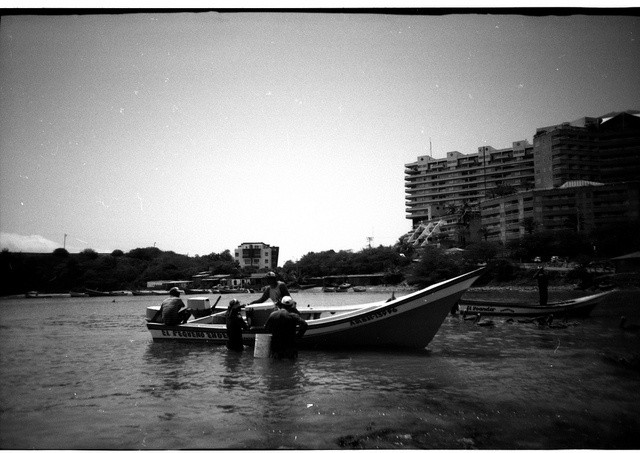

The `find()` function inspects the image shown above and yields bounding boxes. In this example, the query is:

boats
[447,287,620,321]
[145,263,486,354]
[323,282,366,291]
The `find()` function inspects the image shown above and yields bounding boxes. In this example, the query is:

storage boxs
[187,298,210,311]
[245,305,278,326]
[146,306,162,321]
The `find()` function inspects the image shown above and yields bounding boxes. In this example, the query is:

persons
[161,287,191,327]
[264,296,308,362]
[247,271,301,315]
[224,300,250,351]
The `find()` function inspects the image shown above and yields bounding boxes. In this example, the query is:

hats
[265,271,276,276]
[229,297,241,307]
[170,286,184,292]
[280,295,293,305]
